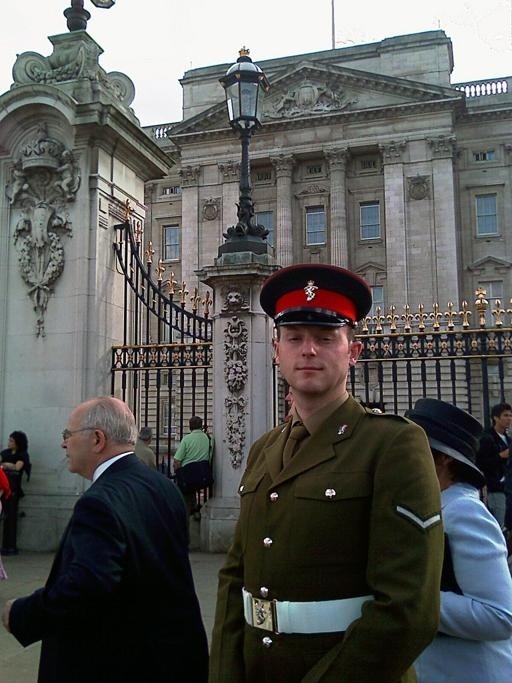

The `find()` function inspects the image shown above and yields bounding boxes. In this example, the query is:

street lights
[219,45,277,257]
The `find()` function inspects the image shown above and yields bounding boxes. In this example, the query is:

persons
[135,427,156,469]
[2,396,210,683]
[285,385,295,407]
[0,467,12,516]
[477,403,512,529]
[174,416,214,515]
[209,263,444,683]
[403,398,512,683]
[0,431,29,555]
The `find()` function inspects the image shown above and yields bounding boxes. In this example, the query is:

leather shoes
[0,548,16,555]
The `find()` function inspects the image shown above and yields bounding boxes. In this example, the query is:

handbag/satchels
[440,533,464,597]
[5,470,24,500]
[175,461,215,494]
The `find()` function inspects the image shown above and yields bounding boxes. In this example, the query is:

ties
[282,425,309,469]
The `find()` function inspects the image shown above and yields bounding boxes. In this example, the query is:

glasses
[63,427,108,442]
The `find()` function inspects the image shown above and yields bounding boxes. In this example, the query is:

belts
[242,587,375,633]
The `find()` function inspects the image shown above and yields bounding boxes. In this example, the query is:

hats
[260,264,372,329]
[404,398,484,476]
